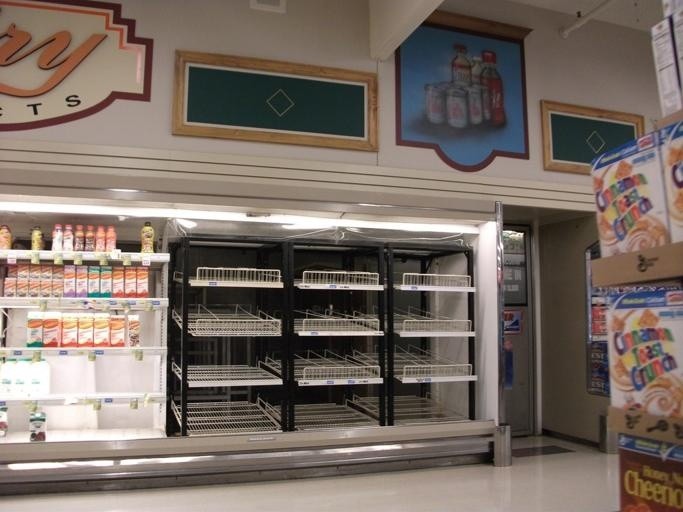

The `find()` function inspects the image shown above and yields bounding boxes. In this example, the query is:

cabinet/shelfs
[289,239,385,431]
[0,250,170,463]
[168,234,288,436]
[385,242,475,426]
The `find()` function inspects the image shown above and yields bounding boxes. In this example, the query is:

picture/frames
[172,49,378,151]
[541,100,644,175]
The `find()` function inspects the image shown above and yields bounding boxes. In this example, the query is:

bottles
[0,219,155,254]
[452,42,506,129]
